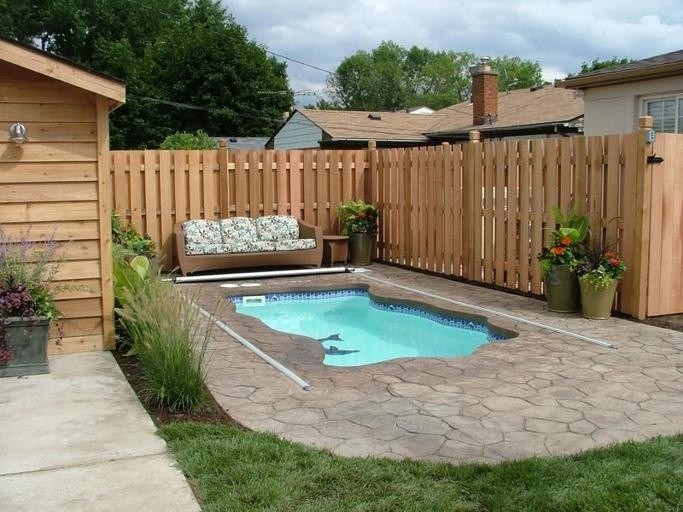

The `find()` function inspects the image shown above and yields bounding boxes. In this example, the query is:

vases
[0,314,52,378]
[347,230,374,264]
[544,258,617,320]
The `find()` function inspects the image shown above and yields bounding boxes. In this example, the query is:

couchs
[173,216,325,275]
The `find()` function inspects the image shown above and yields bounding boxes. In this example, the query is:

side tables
[323,235,350,267]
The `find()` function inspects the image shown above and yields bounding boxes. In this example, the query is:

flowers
[338,198,380,234]
[0,226,69,360]
[538,197,626,289]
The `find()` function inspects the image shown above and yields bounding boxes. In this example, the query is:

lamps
[7,123,27,144]
